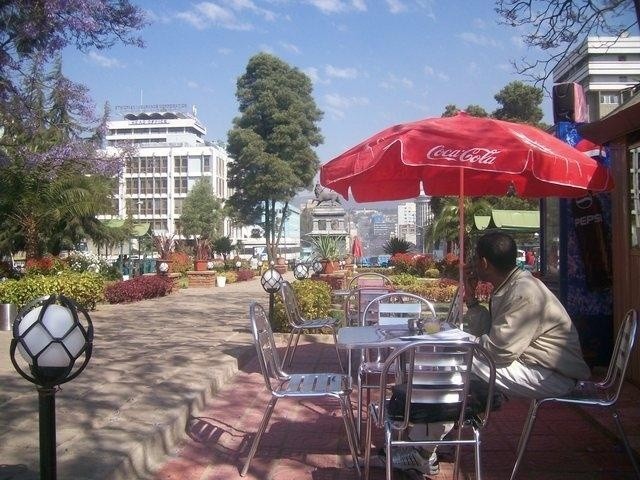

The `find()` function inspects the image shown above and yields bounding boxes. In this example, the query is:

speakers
[552,81,585,124]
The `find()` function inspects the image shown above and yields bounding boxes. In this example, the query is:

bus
[213,236,302,269]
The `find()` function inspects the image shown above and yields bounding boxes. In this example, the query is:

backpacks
[388,369,505,422]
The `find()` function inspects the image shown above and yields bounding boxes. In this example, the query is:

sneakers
[377,445,441,476]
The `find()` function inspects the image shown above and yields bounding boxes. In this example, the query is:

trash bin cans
[0,303,16,331]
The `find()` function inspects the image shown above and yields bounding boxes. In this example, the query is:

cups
[407,318,421,331]
[426,317,440,333]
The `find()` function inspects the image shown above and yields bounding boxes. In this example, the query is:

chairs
[347,272,392,290]
[279,280,345,374]
[510,309,637,480]
[364,339,496,480]
[344,287,402,326]
[363,292,437,406]
[239,302,363,478]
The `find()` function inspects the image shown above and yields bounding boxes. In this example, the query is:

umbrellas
[319,111,617,331]
[353,238,362,272]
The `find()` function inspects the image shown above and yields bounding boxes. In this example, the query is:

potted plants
[146,232,179,274]
[301,229,349,274]
[193,238,211,272]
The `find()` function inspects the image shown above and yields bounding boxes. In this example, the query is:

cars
[127,255,138,259]
[357,254,391,269]
[516,249,526,263]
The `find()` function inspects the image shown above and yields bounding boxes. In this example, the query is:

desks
[335,287,388,296]
[339,323,468,467]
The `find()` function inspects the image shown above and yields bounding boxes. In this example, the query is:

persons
[380,233,591,475]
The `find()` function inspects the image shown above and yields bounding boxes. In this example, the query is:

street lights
[110,201,145,219]
[408,222,425,254]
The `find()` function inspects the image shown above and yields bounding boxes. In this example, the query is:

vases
[215,275,227,288]
